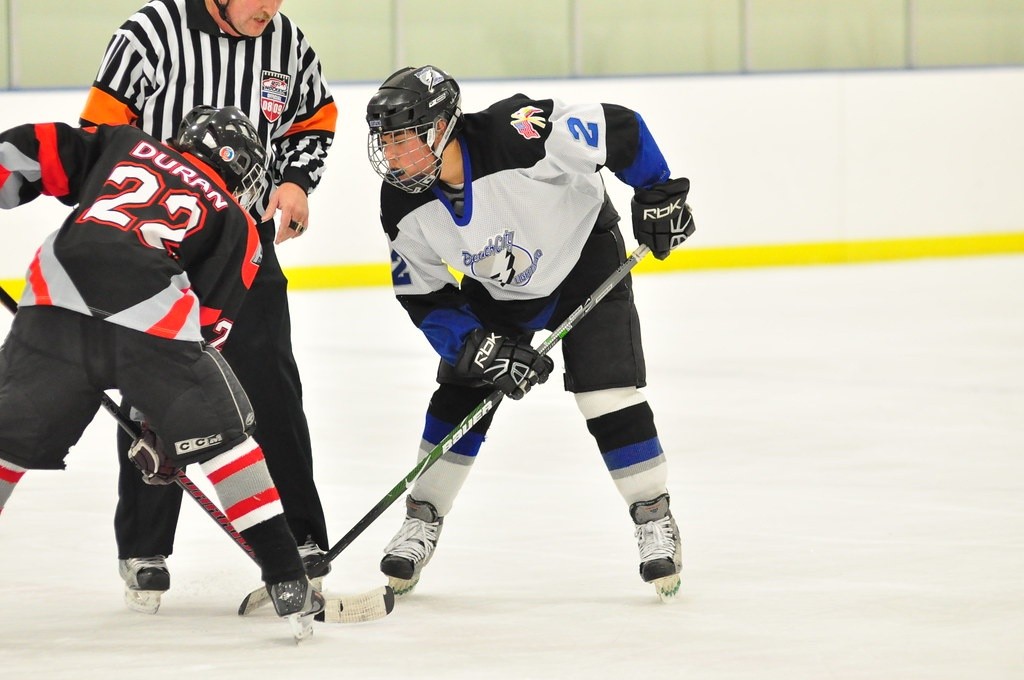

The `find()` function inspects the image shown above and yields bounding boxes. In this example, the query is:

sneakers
[294,537,333,591]
[629,492,683,602]
[263,573,325,642]
[118,551,171,613]
[380,494,444,595]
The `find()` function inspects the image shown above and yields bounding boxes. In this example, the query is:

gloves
[126,426,183,484]
[454,328,555,400]
[630,178,696,261]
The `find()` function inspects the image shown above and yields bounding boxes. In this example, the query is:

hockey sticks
[231,239,660,620]
[0,280,402,630]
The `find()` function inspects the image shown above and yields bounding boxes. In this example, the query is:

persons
[2,0,339,643]
[363,64,696,596]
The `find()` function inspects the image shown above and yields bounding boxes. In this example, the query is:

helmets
[366,65,461,194]
[174,104,267,193]
